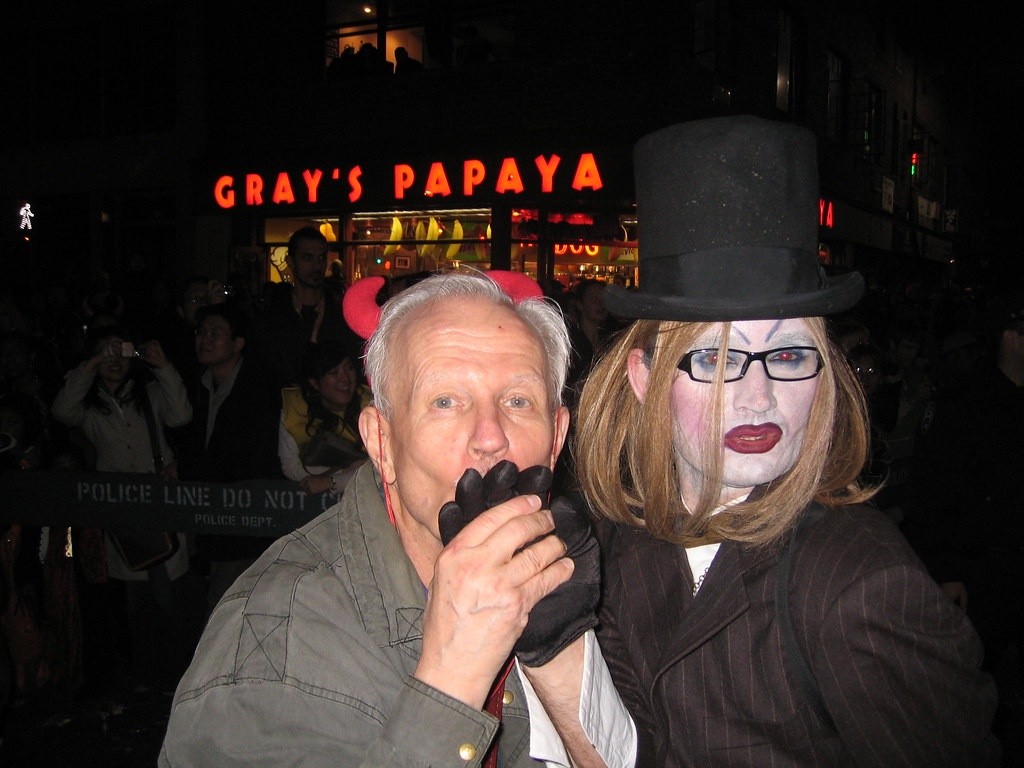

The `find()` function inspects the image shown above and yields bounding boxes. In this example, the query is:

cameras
[121,342,144,356]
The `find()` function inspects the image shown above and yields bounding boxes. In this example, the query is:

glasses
[194,327,233,341]
[188,296,207,304]
[677,346,824,384]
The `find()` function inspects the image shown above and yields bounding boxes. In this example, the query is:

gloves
[438,459,602,668]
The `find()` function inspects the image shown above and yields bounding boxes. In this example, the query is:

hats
[603,115,868,322]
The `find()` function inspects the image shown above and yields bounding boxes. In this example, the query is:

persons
[152,269,572,768]
[438,314,1018,768]
[0,224,1024,768]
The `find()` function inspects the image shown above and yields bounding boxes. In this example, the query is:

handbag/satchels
[0,544,42,666]
[108,527,180,571]
